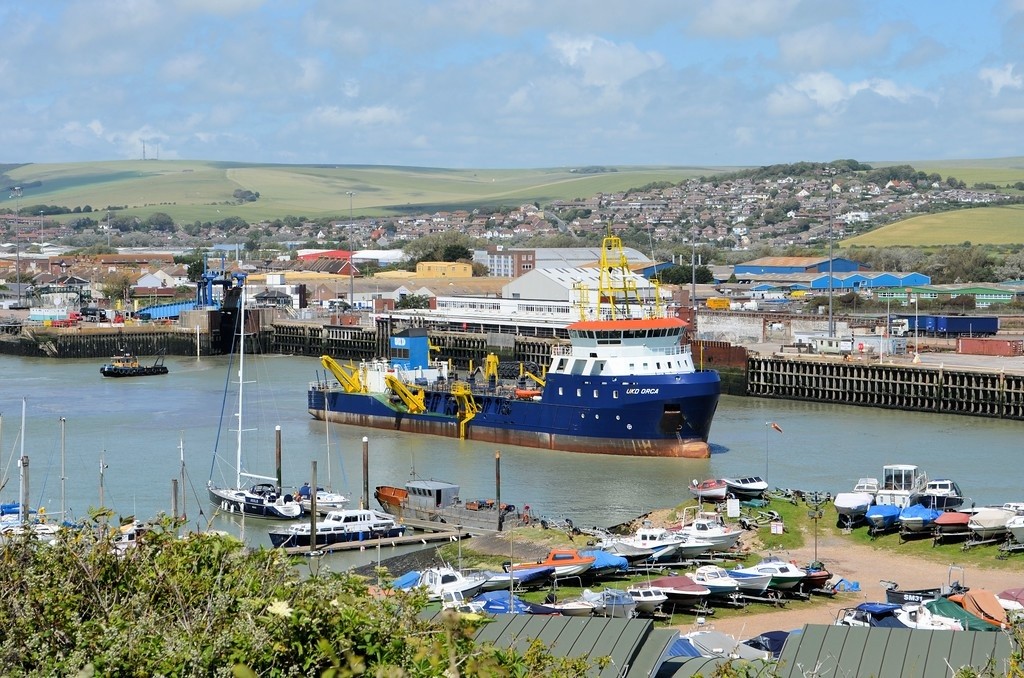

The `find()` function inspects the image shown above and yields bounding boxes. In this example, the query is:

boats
[688,476,727,503]
[347,463,1024,661]
[265,496,410,549]
[725,472,769,497]
[373,452,544,538]
[100,337,169,377]
[515,388,542,399]
[305,192,722,461]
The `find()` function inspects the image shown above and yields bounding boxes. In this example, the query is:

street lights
[9,185,25,307]
[807,511,826,561]
[106,209,112,249]
[345,190,356,309]
[39,210,46,253]
[821,166,842,337]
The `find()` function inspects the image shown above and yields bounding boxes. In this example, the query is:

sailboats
[290,396,351,516]
[0,396,189,562]
[206,284,306,525]
